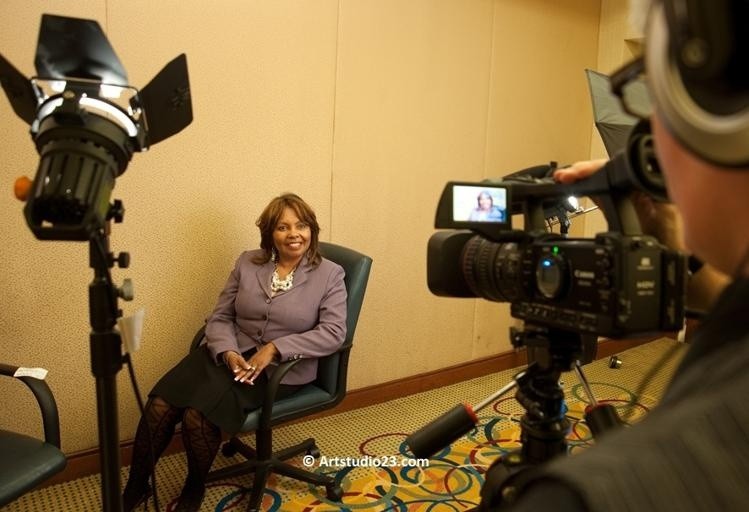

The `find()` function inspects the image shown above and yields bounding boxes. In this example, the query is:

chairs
[191,242,372,512]
[0,362,68,511]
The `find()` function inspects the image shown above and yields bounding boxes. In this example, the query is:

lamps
[0,13,194,512]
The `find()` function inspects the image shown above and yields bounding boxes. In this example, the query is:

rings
[250,367,256,371]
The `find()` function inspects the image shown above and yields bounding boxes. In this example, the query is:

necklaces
[271,260,297,291]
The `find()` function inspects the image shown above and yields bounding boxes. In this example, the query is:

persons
[468,192,502,221]
[502,1,747,509]
[122,192,347,512]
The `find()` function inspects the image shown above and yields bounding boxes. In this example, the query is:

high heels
[124,483,152,511]
